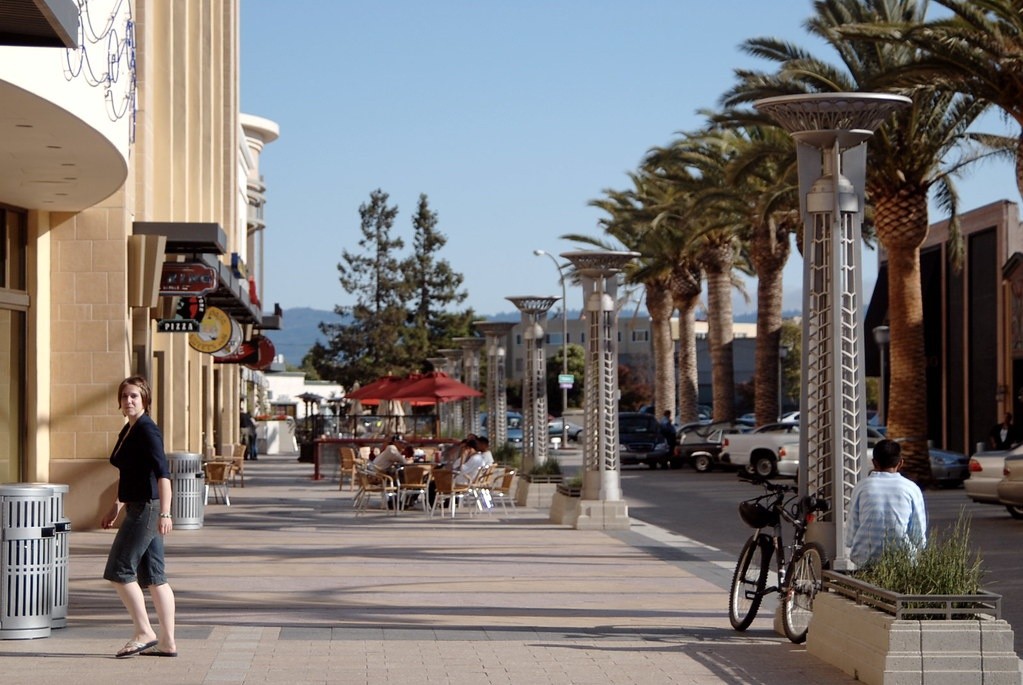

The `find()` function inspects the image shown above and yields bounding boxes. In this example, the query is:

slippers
[115,640,158,657]
[139,645,177,656]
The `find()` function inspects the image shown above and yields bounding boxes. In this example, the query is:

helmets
[740,501,770,529]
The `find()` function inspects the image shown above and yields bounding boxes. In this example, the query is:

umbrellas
[343,369,484,446]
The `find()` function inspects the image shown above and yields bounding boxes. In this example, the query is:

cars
[477,411,585,449]
[617,411,672,471]
[962,441,1023,524]
[641,402,970,491]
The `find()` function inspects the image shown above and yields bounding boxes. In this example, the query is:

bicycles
[729,473,831,644]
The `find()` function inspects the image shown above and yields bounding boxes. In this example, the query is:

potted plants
[804,503,1021,685]
[517,457,565,509]
[549,467,584,524]
[491,441,522,493]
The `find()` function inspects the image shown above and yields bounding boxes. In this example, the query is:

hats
[392,434,406,443]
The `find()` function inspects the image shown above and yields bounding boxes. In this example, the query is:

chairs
[215,444,247,488]
[340,447,519,519]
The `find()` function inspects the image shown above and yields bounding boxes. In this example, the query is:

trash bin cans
[0,480,73,639]
[163,453,207,531]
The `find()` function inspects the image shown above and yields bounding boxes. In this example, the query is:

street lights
[533,248,568,448]
[778,344,789,420]
[872,325,891,428]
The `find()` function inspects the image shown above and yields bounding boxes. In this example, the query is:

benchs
[203,461,232,506]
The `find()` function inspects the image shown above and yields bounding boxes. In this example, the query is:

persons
[240,407,260,460]
[368,432,494,507]
[993,412,1016,451]
[101,377,178,657]
[661,410,675,433]
[844,439,927,570]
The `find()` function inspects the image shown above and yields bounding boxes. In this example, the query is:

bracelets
[159,513,172,518]
